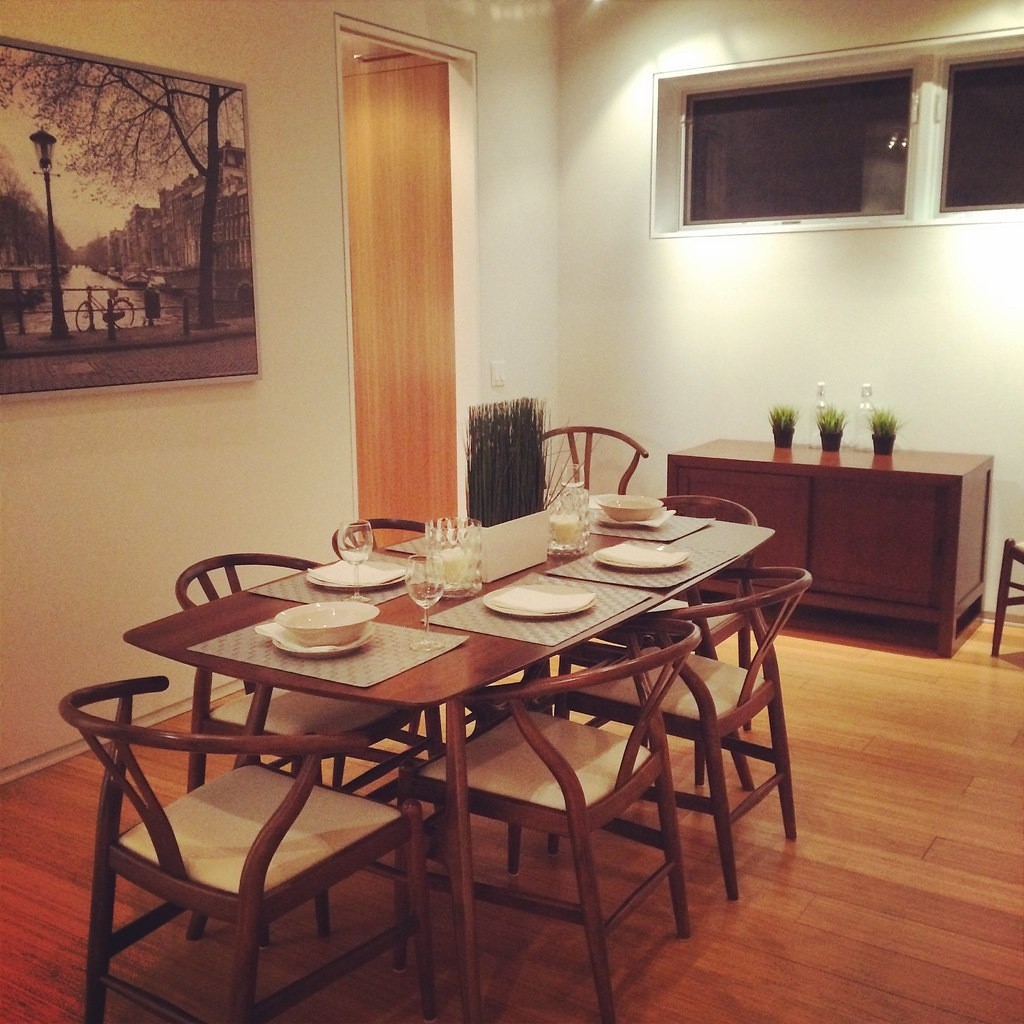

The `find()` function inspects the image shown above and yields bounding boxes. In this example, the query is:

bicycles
[75,282,137,333]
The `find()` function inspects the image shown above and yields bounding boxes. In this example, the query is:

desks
[122,507,776,1024]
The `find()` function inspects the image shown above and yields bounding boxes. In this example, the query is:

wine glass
[405,554,447,651]
[337,518,374,603]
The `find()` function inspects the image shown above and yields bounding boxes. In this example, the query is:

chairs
[992,538,1024,656]
[58,426,813,1024]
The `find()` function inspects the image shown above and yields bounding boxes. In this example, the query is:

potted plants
[768,406,799,448]
[815,406,848,451]
[867,405,902,455]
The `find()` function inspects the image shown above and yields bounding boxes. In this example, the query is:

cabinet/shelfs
[667,438,994,659]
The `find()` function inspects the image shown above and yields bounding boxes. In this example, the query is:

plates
[482,584,598,617]
[307,562,409,589]
[593,541,688,569]
[273,636,373,657]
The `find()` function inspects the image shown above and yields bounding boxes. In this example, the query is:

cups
[541,487,591,557]
[560,465,586,490]
[424,517,484,597]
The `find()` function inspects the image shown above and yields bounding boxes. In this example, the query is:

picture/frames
[0,35,263,401]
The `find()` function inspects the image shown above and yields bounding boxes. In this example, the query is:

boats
[106,262,167,289]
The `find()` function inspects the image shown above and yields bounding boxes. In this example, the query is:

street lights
[29,125,69,340]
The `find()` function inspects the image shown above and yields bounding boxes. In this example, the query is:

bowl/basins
[275,601,379,646]
[595,494,665,521]
